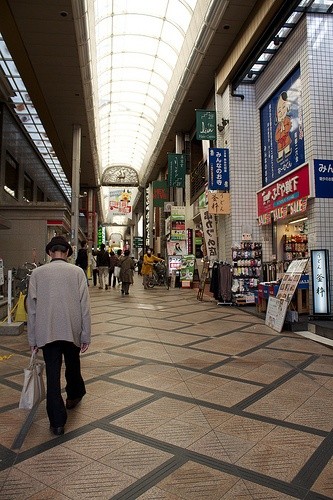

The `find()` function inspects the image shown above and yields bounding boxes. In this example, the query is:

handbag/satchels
[114,266,121,277]
[18,349,46,410]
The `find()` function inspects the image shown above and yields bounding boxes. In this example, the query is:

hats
[45,236,73,257]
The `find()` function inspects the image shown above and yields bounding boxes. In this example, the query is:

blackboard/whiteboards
[199,262,208,290]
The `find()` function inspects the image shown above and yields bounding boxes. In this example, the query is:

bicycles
[4,259,44,298]
[145,261,170,290]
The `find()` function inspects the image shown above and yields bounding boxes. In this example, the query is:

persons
[141,248,165,289]
[78,240,135,296]
[174,243,183,256]
[26,235,91,434]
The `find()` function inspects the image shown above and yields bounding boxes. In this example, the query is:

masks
[147,251,151,254]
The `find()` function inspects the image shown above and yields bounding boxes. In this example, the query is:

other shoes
[99,286,103,289]
[66,396,82,409]
[48,425,65,435]
[144,283,151,289]
[121,290,129,294]
[105,284,108,290]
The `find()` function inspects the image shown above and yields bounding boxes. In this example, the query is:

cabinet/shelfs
[231,234,310,315]
